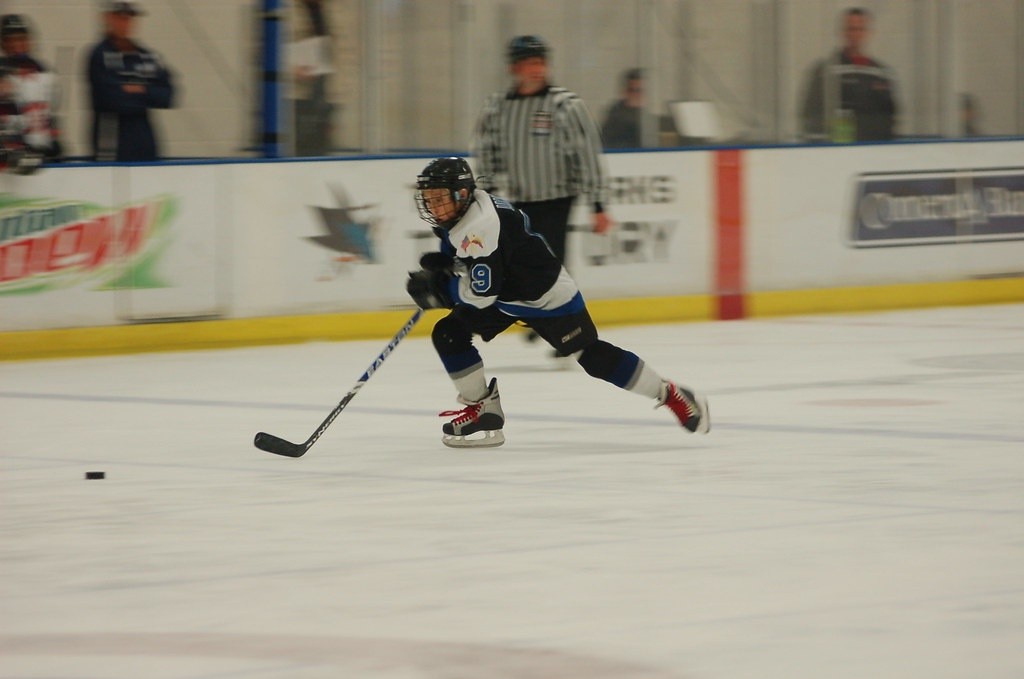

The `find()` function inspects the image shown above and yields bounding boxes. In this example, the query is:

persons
[798,8,896,143]
[602,67,642,154]
[0,15,63,167]
[84,1,174,162]
[407,158,708,447]
[471,36,611,341]
[285,0,335,156]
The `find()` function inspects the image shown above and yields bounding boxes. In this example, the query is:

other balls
[86,471,105,480]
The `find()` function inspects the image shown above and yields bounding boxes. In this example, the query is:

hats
[0,13,27,30]
[99,0,138,17]
[507,34,548,64]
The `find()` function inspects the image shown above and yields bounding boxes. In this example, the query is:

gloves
[418,252,457,276]
[407,271,453,311]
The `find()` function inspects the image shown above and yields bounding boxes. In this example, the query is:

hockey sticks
[254,308,423,459]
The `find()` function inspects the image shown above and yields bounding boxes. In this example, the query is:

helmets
[415,158,476,204]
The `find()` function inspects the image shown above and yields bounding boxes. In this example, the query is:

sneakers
[439,377,505,448]
[654,380,709,433]
